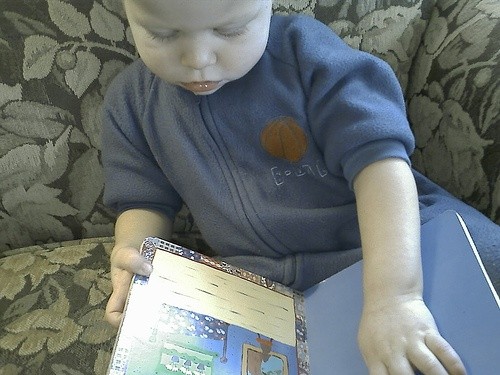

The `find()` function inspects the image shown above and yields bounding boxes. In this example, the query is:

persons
[98,0,500,375]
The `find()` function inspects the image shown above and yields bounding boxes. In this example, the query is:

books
[108,208,499,375]
